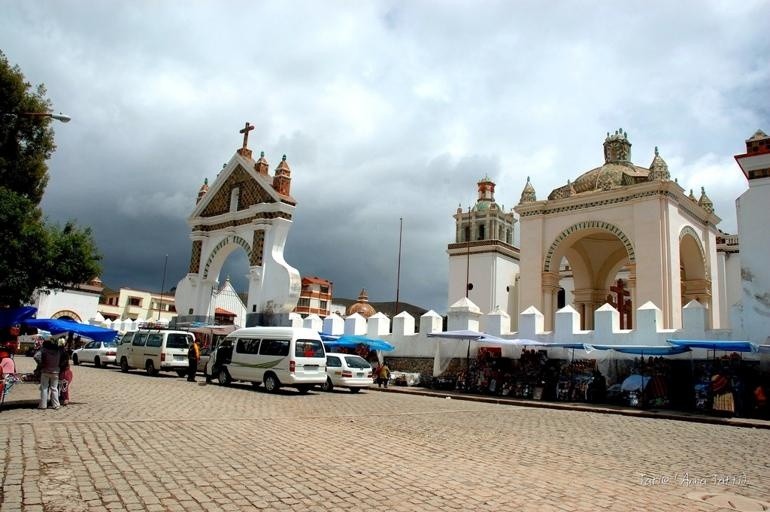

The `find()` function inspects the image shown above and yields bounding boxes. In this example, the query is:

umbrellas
[24,318,119,359]
[178,321,395,351]
[0,305,38,333]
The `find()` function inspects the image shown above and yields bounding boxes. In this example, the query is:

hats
[195,338,200,341]
[1,352,9,358]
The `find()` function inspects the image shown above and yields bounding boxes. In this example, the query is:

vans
[116,328,195,377]
[203,326,328,392]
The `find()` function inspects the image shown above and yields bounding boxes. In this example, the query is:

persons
[187,337,202,382]
[204,346,232,383]
[378,362,391,387]
[32,334,84,409]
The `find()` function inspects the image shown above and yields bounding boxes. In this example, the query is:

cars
[321,352,374,393]
[71,340,119,368]
[197,347,212,372]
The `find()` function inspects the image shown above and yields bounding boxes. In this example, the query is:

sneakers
[187,378,195,381]
[37,400,68,409]
